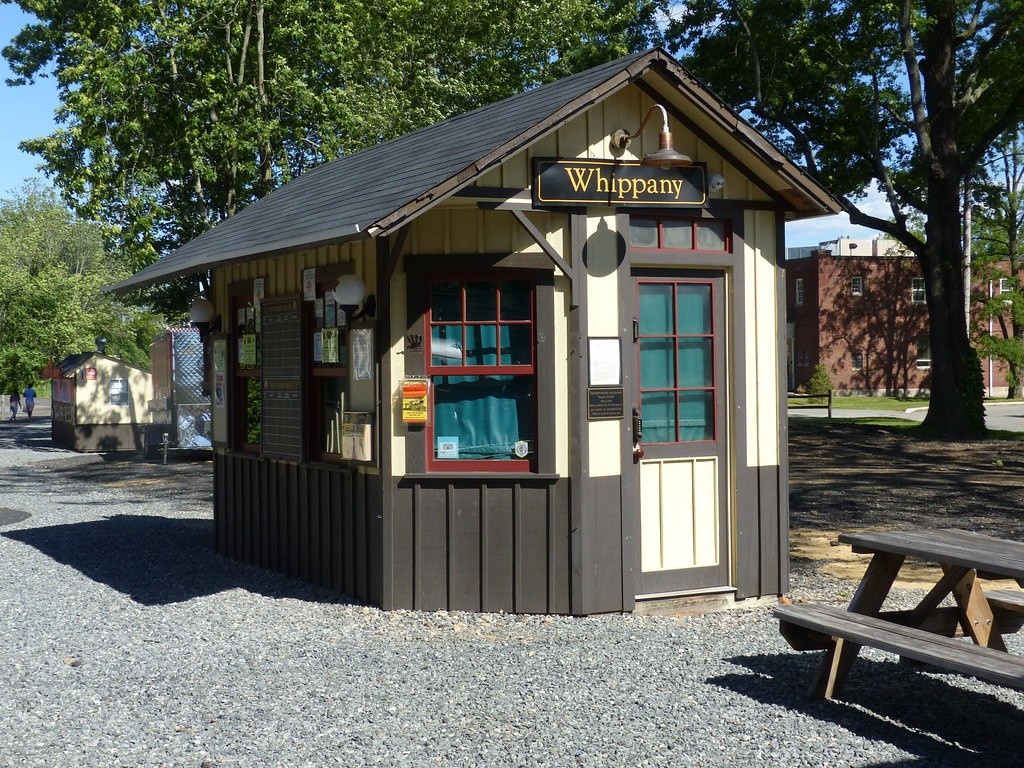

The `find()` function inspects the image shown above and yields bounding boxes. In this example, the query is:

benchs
[772,602,1024,691]
[983,588,1024,634]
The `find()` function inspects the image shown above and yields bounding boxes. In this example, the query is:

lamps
[190,299,222,343]
[332,275,375,332]
[611,104,693,170]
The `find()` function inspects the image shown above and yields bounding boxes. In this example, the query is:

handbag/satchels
[22,406,28,413]
[10,401,17,409]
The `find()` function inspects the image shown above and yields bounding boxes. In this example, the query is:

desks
[804,528,1024,700]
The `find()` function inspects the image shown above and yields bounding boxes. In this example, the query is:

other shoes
[9,419,17,422]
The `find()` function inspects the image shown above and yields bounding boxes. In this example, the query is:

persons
[9,390,21,422]
[24,384,36,418]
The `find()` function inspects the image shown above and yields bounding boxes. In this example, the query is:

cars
[916,359,931,371]
